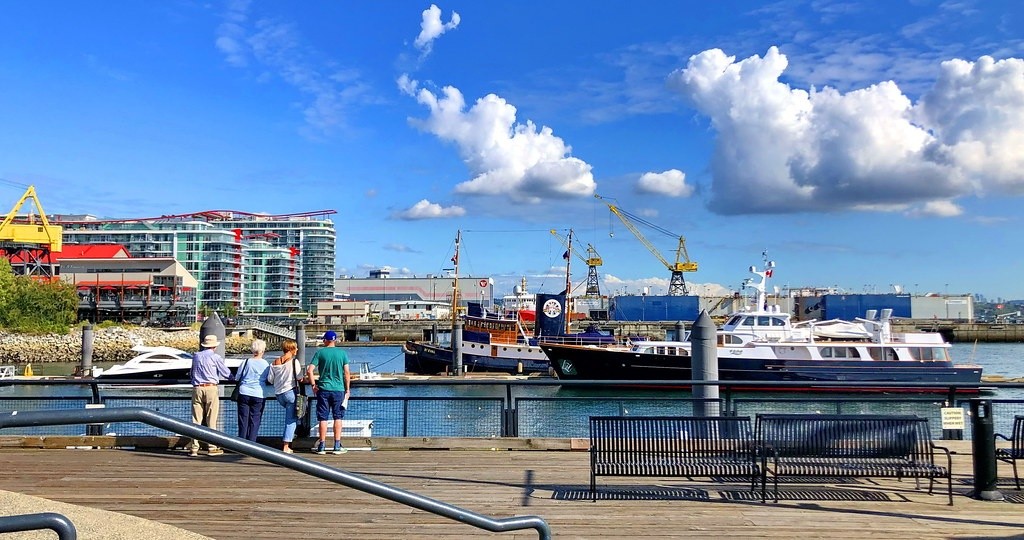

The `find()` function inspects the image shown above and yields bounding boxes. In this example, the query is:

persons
[235,339,269,457]
[188,335,234,457]
[306,330,351,455]
[267,340,309,455]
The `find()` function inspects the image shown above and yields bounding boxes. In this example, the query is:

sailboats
[403,229,551,375]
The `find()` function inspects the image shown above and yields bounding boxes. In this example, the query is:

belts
[194,383,216,387]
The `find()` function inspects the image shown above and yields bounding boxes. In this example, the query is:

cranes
[550,229,603,296]
[594,195,698,296]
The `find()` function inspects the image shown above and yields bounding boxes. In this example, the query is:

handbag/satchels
[231,379,240,401]
[296,394,307,419]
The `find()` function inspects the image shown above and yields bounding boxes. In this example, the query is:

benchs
[588,416,765,504]
[994,414,1024,491]
[754,414,953,504]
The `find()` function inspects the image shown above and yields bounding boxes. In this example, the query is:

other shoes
[314,445,326,454]
[332,444,348,455]
[283,448,293,455]
[188,448,198,456]
[207,449,224,456]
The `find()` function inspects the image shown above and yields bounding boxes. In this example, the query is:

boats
[70,330,280,384]
[981,377,1024,387]
[534,249,999,394]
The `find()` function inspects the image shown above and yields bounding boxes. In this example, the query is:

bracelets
[311,384,316,387]
[346,387,350,390]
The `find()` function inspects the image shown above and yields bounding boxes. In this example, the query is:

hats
[321,331,337,340]
[201,334,220,347]
